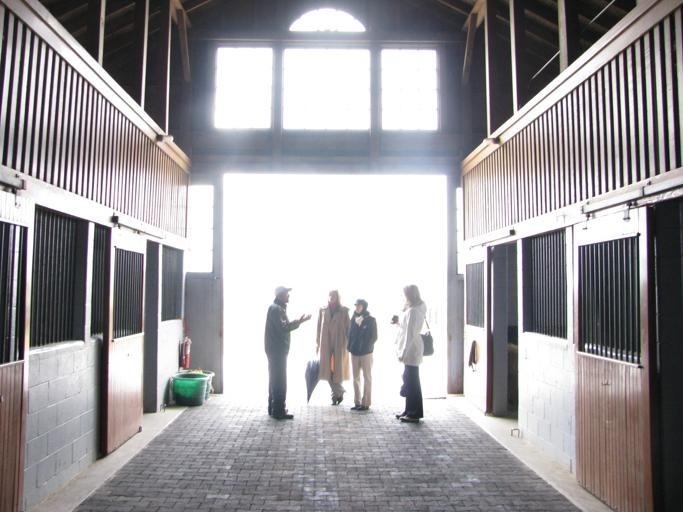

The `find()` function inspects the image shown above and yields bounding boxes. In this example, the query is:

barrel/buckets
[201,370,215,400]
[172,374,209,406]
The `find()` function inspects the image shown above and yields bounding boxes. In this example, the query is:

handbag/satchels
[419,330,433,355]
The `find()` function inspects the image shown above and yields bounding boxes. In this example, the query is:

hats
[354,300,368,307]
[275,286,292,295]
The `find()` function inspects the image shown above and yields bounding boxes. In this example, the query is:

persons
[343,299,379,411]
[390,284,427,424]
[314,290,352,408]
[263,286,312,421]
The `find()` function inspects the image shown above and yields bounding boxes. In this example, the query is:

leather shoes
[351,405,369,410]
[338,390,345,404]
[333,400,338,405]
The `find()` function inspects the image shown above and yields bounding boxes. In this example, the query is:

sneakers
[400,416,419,422]
[273,409,293,418]
[396,412,406,417]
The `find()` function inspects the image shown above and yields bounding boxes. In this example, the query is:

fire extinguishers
[180,336,192,368]
